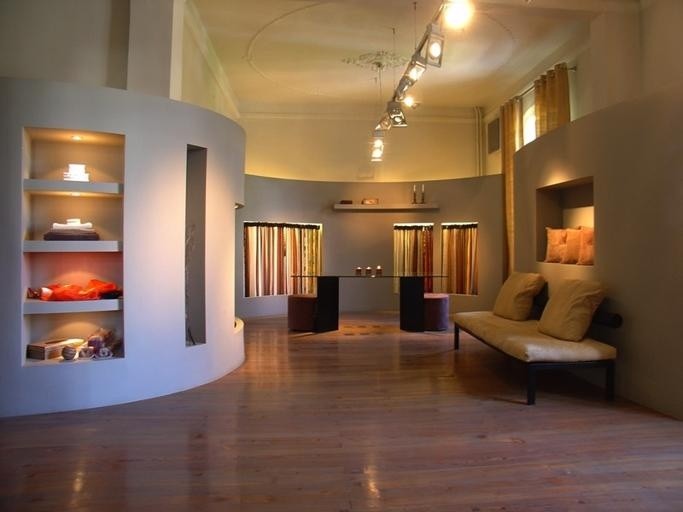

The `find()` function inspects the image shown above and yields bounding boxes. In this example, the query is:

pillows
[544,225,581,263]
[576,226,593,265]
[493,272,545,320]
[561,229,582,263]
[538,278,609,341]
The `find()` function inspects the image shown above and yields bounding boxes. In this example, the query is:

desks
[291,276,448,334]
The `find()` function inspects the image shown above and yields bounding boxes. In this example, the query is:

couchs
[449,312,622,406]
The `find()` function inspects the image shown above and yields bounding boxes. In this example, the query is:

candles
[414,185,416,192]
[422,184,424,193]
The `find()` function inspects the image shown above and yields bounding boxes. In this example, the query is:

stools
[288,294,317,331]
[424,293,448,332]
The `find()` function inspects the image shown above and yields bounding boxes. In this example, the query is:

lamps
[371,129,386,162]
[424,21,445,68]
[395,89,422,110]
[401,55,427,87]
[386,101,408,128]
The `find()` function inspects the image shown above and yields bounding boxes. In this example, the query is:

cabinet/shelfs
[236,173,506,320]
[20,126,126,367]
